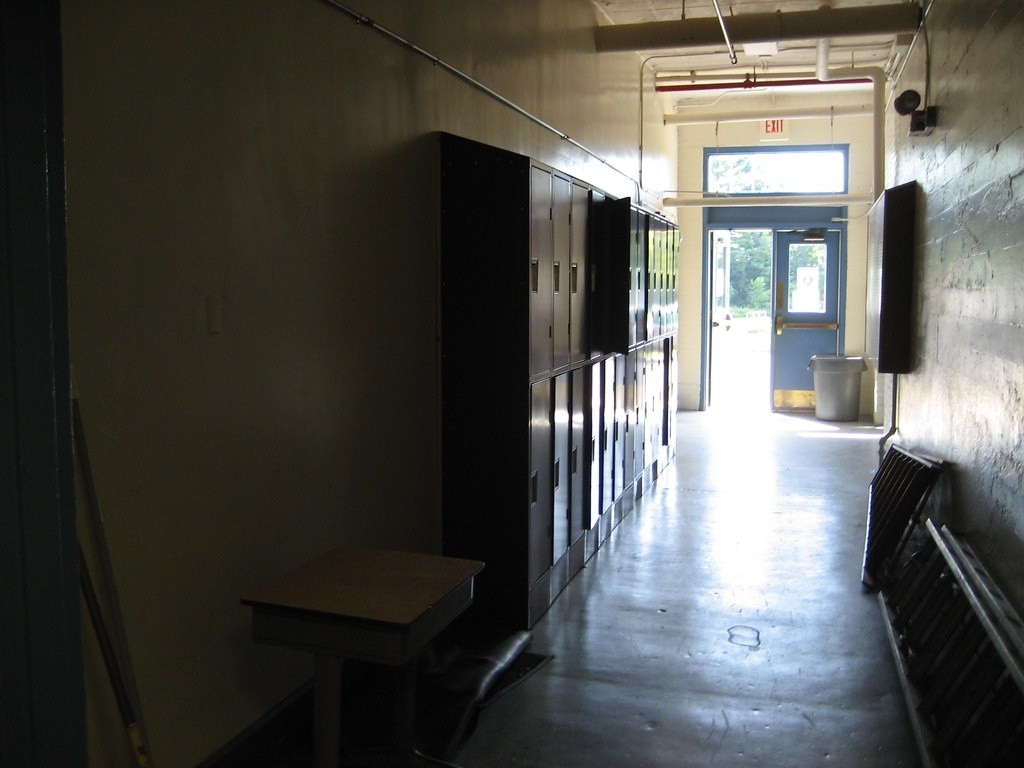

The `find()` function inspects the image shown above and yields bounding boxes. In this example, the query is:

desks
[241,545,486,768]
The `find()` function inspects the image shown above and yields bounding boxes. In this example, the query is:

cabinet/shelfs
[863,179,916,375]
[436,128,682,632]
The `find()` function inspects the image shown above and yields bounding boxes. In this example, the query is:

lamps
[893,89,937,138]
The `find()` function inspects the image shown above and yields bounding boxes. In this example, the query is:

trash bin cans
[807,355,869,422]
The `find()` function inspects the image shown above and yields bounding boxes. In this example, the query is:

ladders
[860,444,955,597]
[877,518,1024,768]
